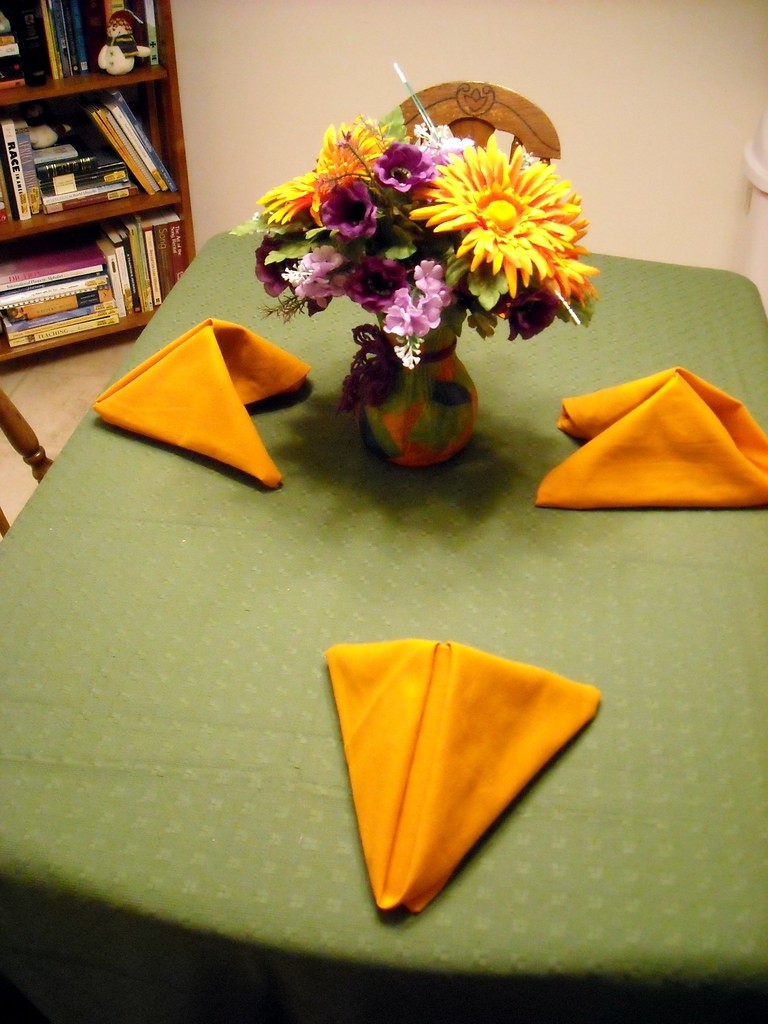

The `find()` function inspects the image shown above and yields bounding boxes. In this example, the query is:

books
[0,208,190,348]
[0,88,178,225]
[0,0,158,89]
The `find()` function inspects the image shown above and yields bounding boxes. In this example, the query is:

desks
[0,231,768,1024]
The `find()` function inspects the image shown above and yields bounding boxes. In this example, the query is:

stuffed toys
[98,10,151,76]
[27,123,76,150]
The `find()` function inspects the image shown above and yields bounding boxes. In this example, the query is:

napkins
[93,318,312,488]
[324,638,603,912]
[535,366,768,510]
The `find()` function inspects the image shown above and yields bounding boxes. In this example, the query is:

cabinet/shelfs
[0,0,196,361]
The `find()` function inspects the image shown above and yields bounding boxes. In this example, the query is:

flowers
[229,105,601,370]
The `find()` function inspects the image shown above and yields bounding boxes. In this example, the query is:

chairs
[400,81,561,166]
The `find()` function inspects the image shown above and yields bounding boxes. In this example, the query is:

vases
[362,311,477,467]
